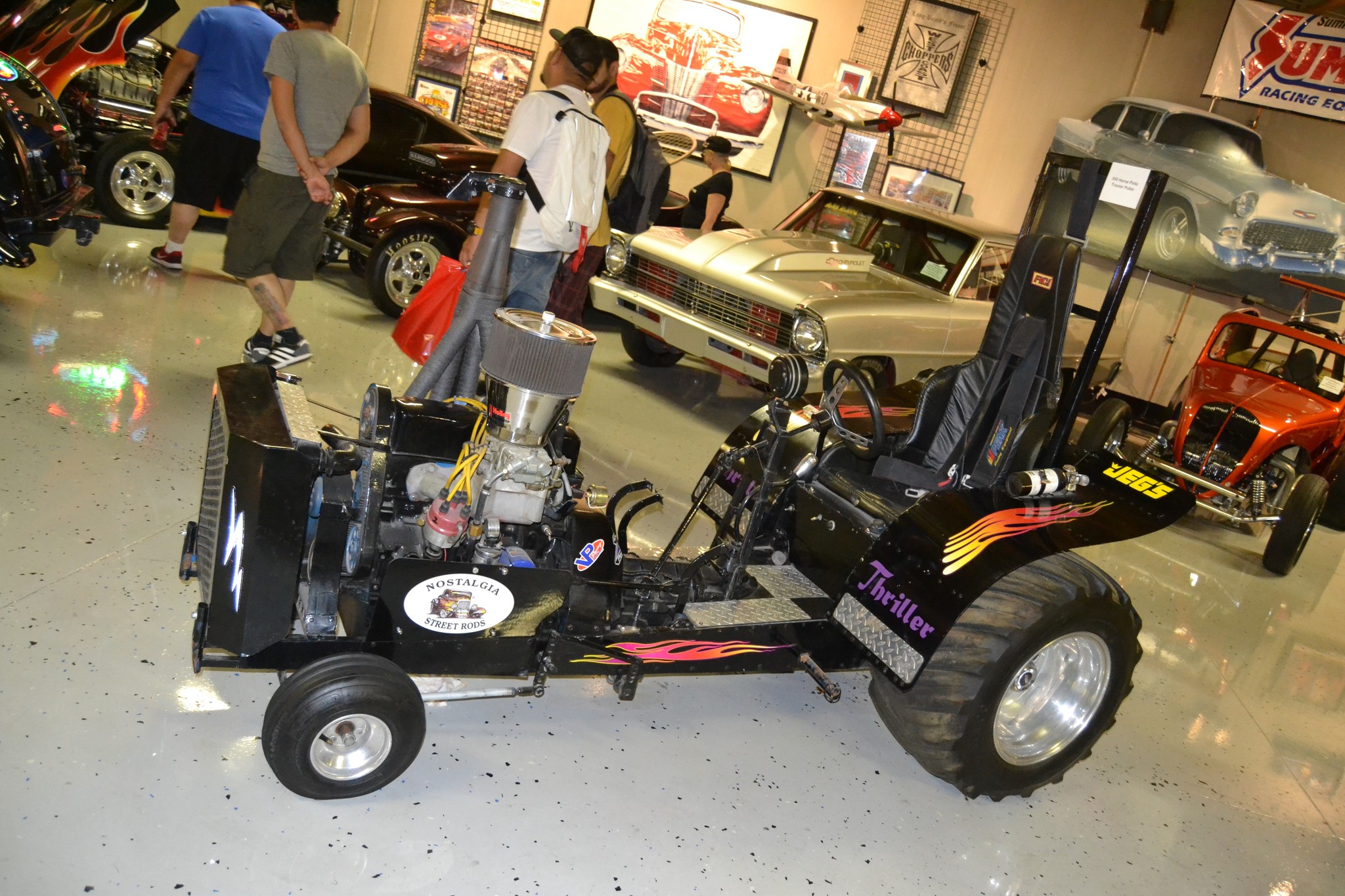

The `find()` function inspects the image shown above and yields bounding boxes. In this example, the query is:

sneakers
[244,333,271,363]
[149,243,183,270]
[256,338,312,369]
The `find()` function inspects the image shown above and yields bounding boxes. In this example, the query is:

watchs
[465,219,485,236]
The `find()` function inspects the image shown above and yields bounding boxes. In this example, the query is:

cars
[1076,270,1344,575]
[178,165,1172,806]
[1051,93,1345,282]
[0,0,747,321]
[609,0,776,168]
[589,182,1125,404]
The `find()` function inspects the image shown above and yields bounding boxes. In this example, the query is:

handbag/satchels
[388,256,474,368]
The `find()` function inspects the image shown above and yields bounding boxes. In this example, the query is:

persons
[147,0,291,281]
[681,136,733,230]
[222,0,372,370]
[456,25,610,311]
[546,37,636,329]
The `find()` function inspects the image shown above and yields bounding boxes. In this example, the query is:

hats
[549,26,602,78]
[702,136,732,154]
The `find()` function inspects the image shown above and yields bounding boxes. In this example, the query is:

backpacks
[591,90,672,235]
